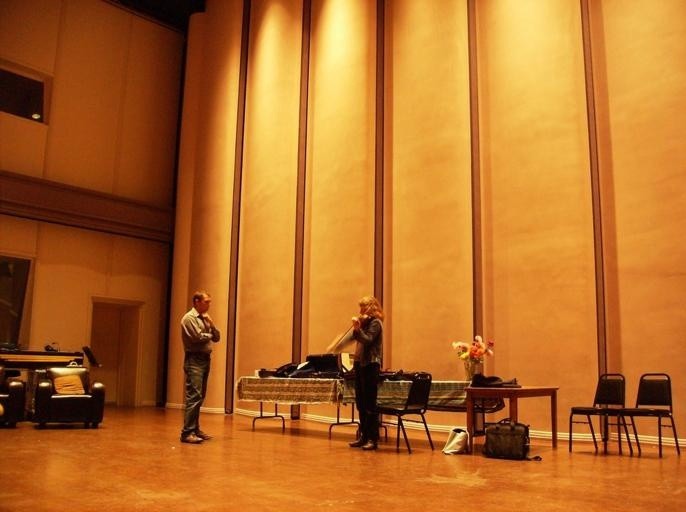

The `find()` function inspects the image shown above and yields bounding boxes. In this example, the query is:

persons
[326,296,386,452]
[179,289,221,444]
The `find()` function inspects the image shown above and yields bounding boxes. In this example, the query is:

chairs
[33,367,105,429]
[612,373,681,458]
[568,374,632,454]
[0,363,24,432]
[377,372,435,454]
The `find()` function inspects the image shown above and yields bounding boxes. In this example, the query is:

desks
[342,379,506,444]
[461,386,559,453]
[236,374,359,439]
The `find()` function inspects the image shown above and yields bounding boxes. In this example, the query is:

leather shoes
[349,436,369,447]
[196,429,212,440]
[179,433,202,443]
[363,439,379,449]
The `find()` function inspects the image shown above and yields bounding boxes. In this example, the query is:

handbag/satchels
[442,428,471,455]
[485,418,530,460]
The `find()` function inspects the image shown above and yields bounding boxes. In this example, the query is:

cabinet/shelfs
[0,352,84,420]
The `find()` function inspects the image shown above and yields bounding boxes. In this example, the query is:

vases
[470,361,483,376]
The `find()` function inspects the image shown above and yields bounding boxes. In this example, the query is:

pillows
[53,375,86,395]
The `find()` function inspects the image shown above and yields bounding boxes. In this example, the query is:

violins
[359,313,371,327]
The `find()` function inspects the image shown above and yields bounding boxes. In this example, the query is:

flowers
[449,334,496,362]
